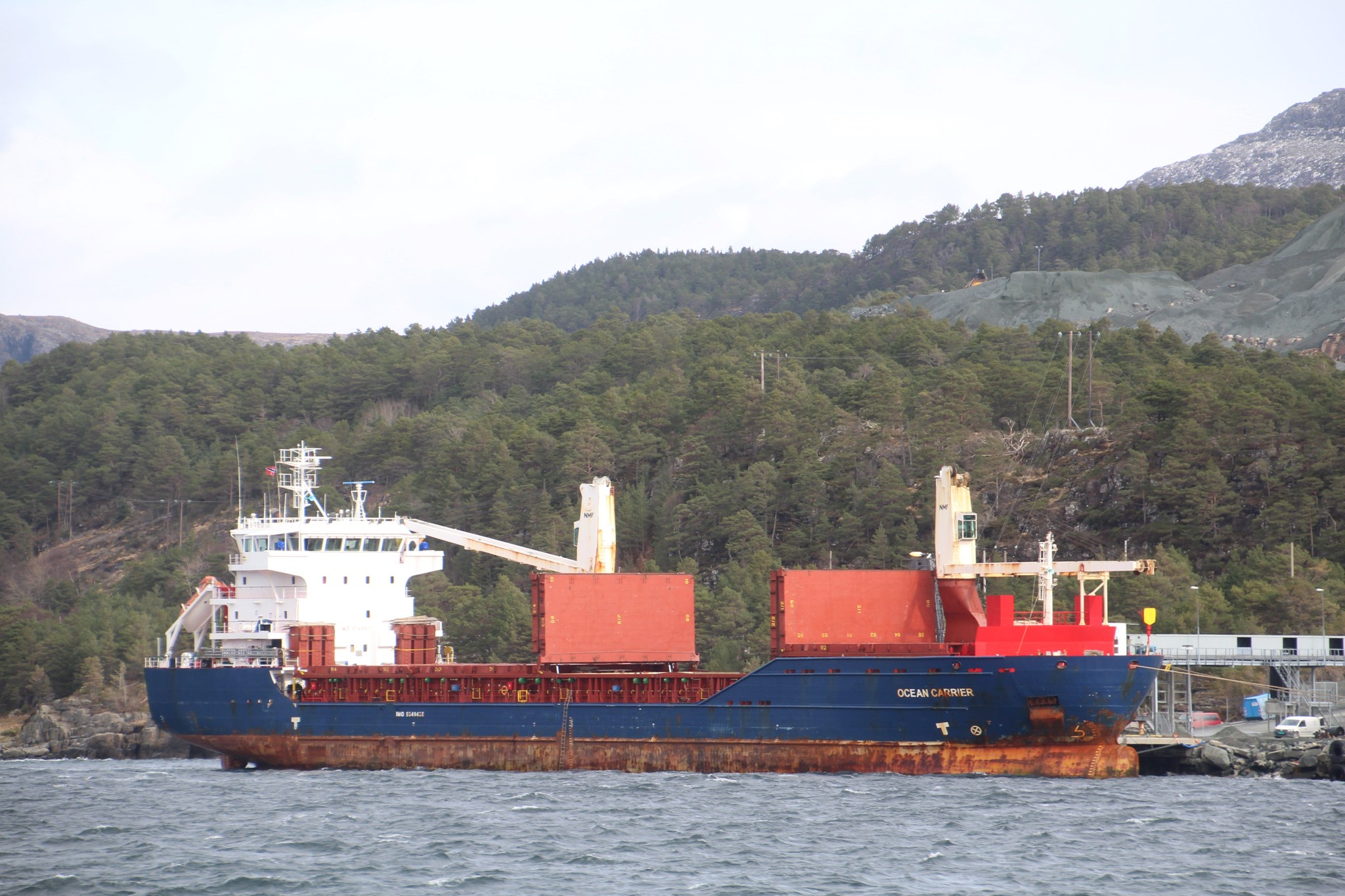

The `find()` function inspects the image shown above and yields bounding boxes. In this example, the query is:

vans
[1273,716,1321,740]
[1179,713,1221,729]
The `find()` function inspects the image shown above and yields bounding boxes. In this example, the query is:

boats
[142,435,1165,786]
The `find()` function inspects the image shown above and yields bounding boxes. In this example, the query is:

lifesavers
[501,686,508,696]
[1329,739,1345,765]
[1165,663,1171,672]
[1329,763,1345,782]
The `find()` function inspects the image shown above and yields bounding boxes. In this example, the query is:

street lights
[1190,585,1200,665]
[1315,588,1327,665]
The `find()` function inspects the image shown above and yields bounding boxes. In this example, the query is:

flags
[266,466,275,476]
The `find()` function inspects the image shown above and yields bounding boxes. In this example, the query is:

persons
[296,682,303,701]
[1314,730,1323,738]
